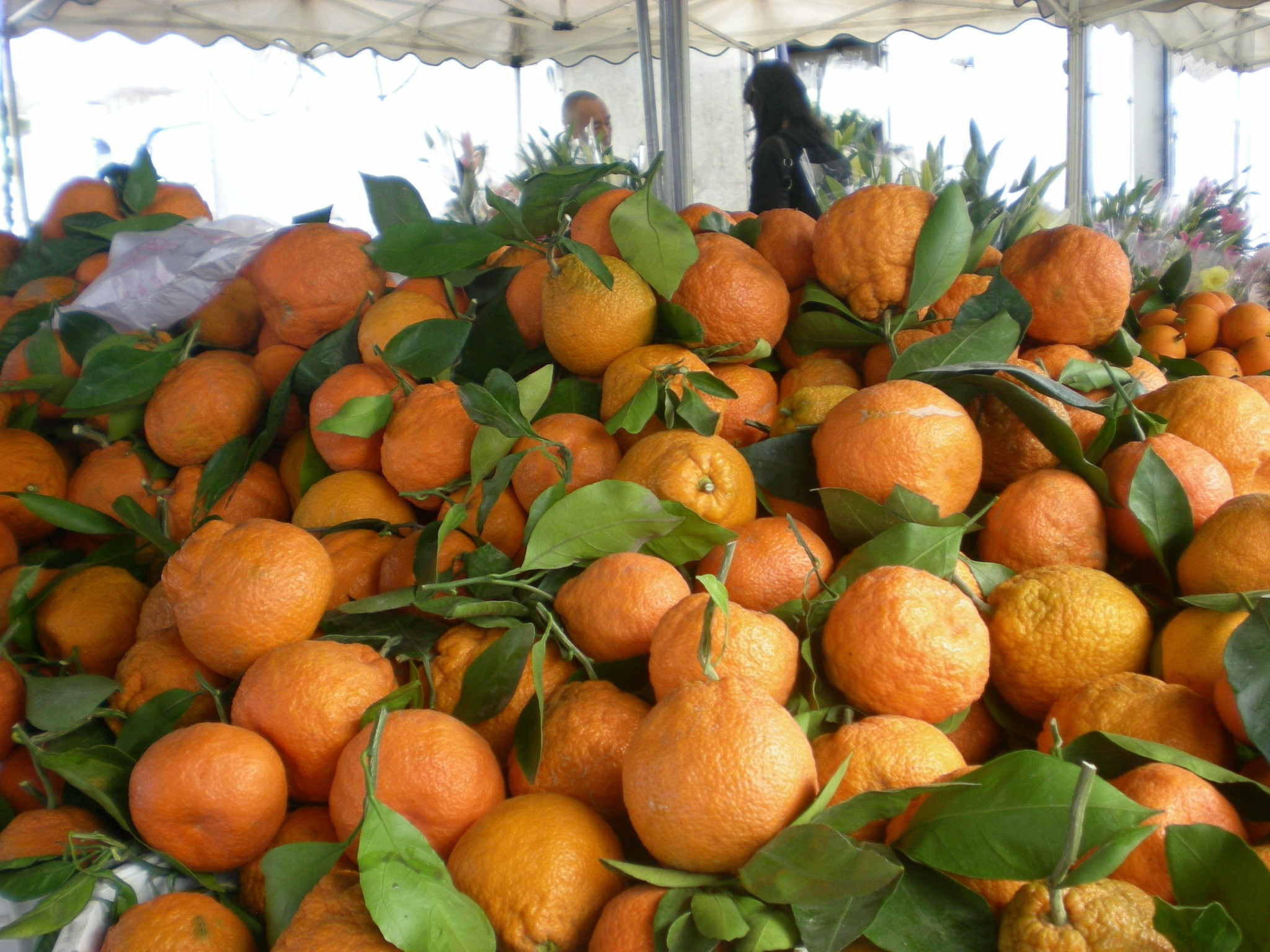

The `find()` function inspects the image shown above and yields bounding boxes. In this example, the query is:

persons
[521,89,637,177]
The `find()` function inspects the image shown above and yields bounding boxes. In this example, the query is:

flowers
[796,118,1071,253]
[419,116,615,227]
[1093,164,1270,301]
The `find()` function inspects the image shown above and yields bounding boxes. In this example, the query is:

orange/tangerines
[0,177,1269,951]
